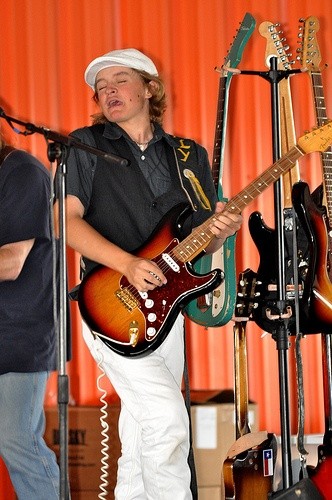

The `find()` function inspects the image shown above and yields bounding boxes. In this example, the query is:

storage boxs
[43,406,123,500]
[181,389,259,500]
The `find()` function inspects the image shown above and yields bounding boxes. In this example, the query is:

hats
[84,47,159,92]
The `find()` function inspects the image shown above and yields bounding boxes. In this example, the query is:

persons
[0,136,70,500]
[53,48,245,500]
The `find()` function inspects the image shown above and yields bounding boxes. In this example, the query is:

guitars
[221,269,279,500]
[249,16,332,335]
[78,122,331,360]
[180,12,255,328]
